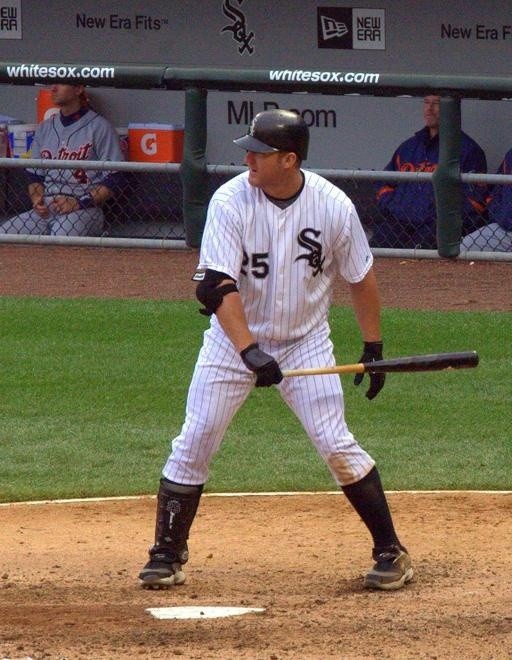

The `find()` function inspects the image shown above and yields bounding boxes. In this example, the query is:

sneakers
[361,542,414,590]
[134,545,189,590]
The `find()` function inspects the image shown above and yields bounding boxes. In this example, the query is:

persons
[367,95,490,249]
[138,109,417,593]
[0,83,128,236]
[457,147,511,252]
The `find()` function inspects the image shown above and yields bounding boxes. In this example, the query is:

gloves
[240,340,284,388]
[353,338,387,401]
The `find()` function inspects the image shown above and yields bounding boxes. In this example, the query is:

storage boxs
[126,122,186,164]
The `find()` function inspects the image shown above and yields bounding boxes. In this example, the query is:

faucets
[5,123,41,158]
[35,87,60,124]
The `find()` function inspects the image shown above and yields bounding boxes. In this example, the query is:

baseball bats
[283,352,478,375]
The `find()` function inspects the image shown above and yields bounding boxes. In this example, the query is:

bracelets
[76,192,96,210]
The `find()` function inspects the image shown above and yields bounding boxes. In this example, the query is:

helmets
[232,108,310,160]
